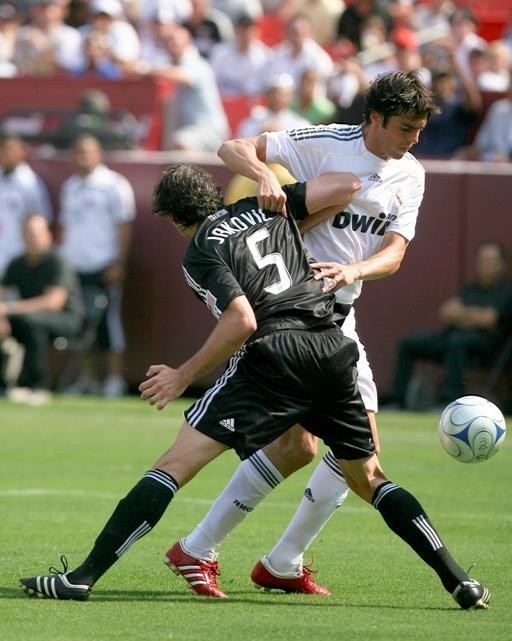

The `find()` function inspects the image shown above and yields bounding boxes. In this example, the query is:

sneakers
[450,579,490,611]
[16,556,94,601]
[161,537,228,600]
[252,555,332,598]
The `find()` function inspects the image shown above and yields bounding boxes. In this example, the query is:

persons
[21,165,492,612]
[164,70,433,599]
[1,1,512,408]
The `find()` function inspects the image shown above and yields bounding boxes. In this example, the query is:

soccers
[438,396,506,464]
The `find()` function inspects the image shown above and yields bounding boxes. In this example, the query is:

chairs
[48,283,109,396]
[405,306,511,414]
